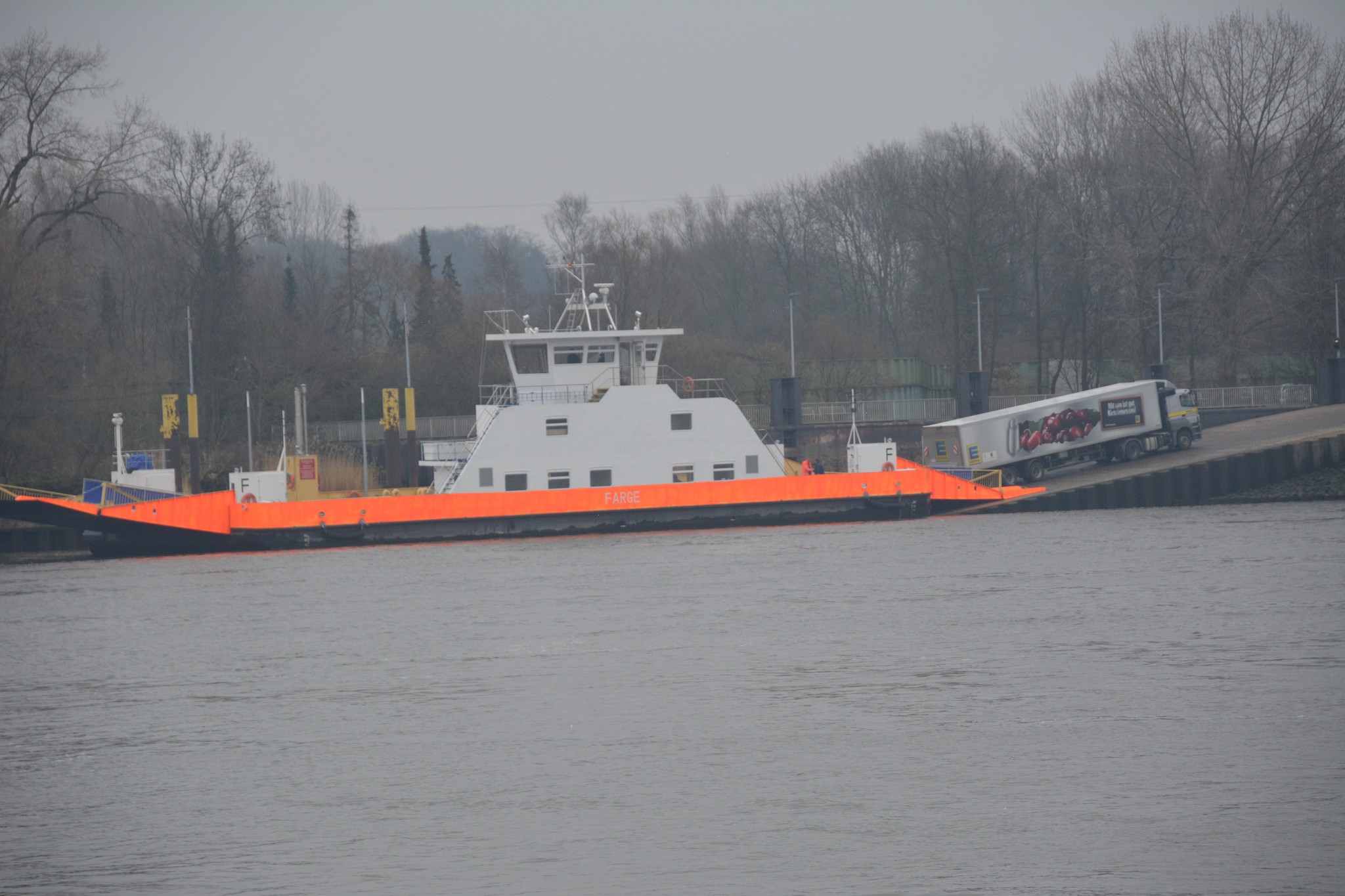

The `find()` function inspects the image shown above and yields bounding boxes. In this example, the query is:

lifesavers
[286,471,294,489]
[416,487,423,495]
[420,487,428,495]
[683,376,695,393]
[391,489,402,496]
[241,493,257,503]
[348,491,361,498]
[882,461,894,472]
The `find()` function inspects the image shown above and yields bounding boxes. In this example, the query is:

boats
[0,254,1048,552]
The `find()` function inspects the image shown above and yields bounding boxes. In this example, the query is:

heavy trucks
[922,378,1204,486]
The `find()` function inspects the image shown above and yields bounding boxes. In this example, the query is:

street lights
[789,292,802,376]
[975,287,992,373]
[1157,282,1171,365]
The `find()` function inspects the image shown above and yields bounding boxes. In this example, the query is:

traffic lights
[1334,336,1342,349]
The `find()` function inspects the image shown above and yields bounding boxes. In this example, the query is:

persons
[812,458,824,474]
[801,457,812,475]
[567,347,581,363]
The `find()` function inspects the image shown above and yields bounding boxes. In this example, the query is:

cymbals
[382,490,391,496]
